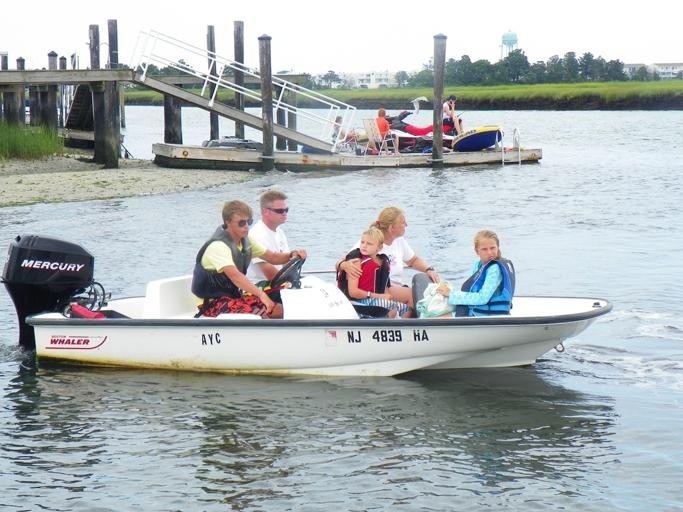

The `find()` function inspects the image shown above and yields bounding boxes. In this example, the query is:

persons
[435,229,515,318]
[246,189,297,298]
[373,108,400,156]
[332,115,356,145]
[334,206,440,318]
[190,199,308,320]
[442,94,464,136]
[337,227,411,320]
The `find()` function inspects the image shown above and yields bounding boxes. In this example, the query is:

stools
[342,135,356,153]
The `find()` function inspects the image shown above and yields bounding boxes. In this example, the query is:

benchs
[144,270,200,318]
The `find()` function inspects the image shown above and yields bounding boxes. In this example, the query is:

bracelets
[367,291,370,298]
[290,249,297,257]
[425,266,435,272]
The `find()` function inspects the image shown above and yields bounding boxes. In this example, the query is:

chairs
[360,118,397,156]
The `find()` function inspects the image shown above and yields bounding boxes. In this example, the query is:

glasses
[267,207,288,213]
[235,218,253,226]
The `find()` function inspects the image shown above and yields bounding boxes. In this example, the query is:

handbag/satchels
[417,281,457,317]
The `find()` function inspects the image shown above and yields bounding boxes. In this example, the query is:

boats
[368,110,462,152]
[0,234,613,377]
[449,126,504,152]
[339,129,368,146]
[57,129,124,150]
